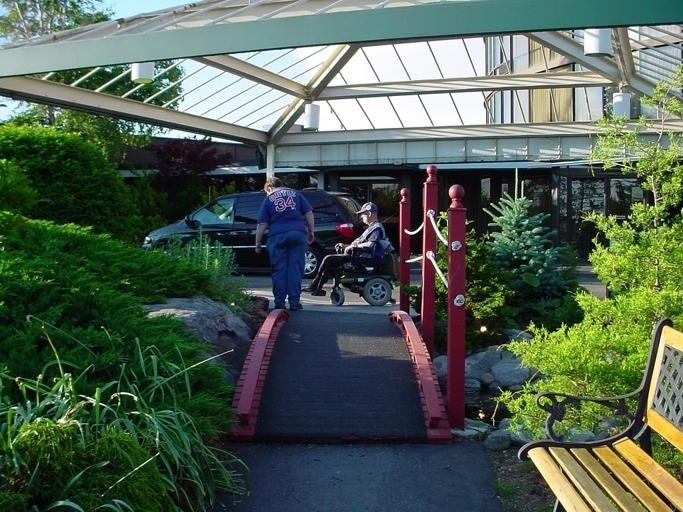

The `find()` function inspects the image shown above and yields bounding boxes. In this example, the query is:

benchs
[518,321,682,512]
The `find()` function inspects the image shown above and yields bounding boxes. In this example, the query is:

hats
[356,202,377,214]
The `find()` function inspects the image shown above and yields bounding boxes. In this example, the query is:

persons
[255,177,315,311]
[301,201,386,294]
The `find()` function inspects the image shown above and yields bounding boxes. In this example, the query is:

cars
[140,187,368,279]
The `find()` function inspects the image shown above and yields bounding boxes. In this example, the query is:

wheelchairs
[310,244,399,307]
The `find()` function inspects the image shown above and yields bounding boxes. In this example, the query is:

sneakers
[302,287,324,296]
[274,303,303,310]
